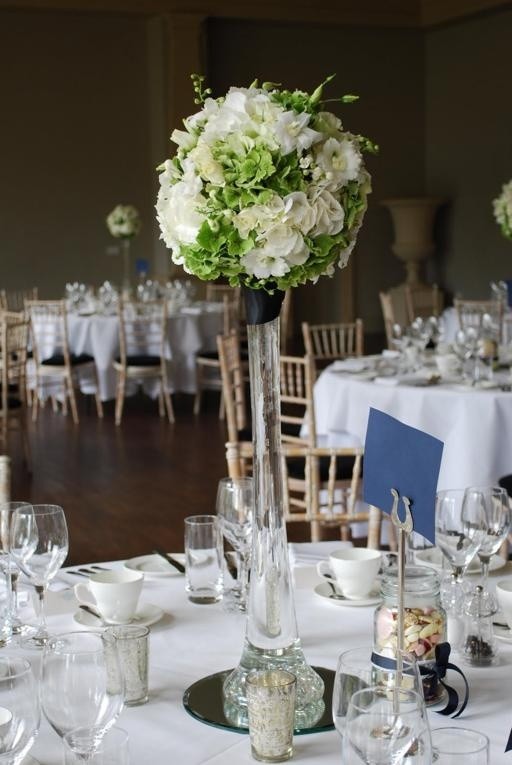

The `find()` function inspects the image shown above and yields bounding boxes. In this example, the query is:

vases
[121,238,134,301]
[222,280,327,728]
[381,197,441,285]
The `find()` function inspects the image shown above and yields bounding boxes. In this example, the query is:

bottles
[461,585,497,668]
[370,564,448,704]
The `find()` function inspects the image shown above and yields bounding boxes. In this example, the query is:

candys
[373,602,443,702]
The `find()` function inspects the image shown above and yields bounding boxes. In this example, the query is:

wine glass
[2,501,39,642]
[460,486,511,617]
[218,486,252,614]
[389,312,512,382]
[65,278,198,315]
[214,478,253,598]
[434,489,489,614]
[8,504,68,651]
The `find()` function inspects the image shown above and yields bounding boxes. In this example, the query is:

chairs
[194,292,254,419]
[275,353,384,550]
[217,329,352,541]
[414,285,439,318]
[111,298,175,426]
[379,286,415,349]
[0,285,104,435]
[302,318,366,365]
[456,300,503,347]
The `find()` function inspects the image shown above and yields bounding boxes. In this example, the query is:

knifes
[222,552,238,580]
[153,547,186,573]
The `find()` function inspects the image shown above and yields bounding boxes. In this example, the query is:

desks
[1,541,512,763]
[31,302,224,399]
[440,308,512,365]
[300,353,512,489]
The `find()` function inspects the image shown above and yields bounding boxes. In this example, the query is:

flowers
[108,204,142,238]
[155,66,378,295]
[492,177,512,236]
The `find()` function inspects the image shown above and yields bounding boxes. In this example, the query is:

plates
[73,602,166,628]
[313,577,382,607]
[122,554,185,578]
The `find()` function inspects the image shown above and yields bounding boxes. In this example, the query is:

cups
[62,722,129,762]
[183,515,224,605]
[103,624,149,706]
[343,687,434,764]
[74,569,146,624]
[246,670,297,762]
[331,646,426,764]
[1,654,40,763]
[316,548,381,600]
[40,629,125,760]
[418,727,490,764]
